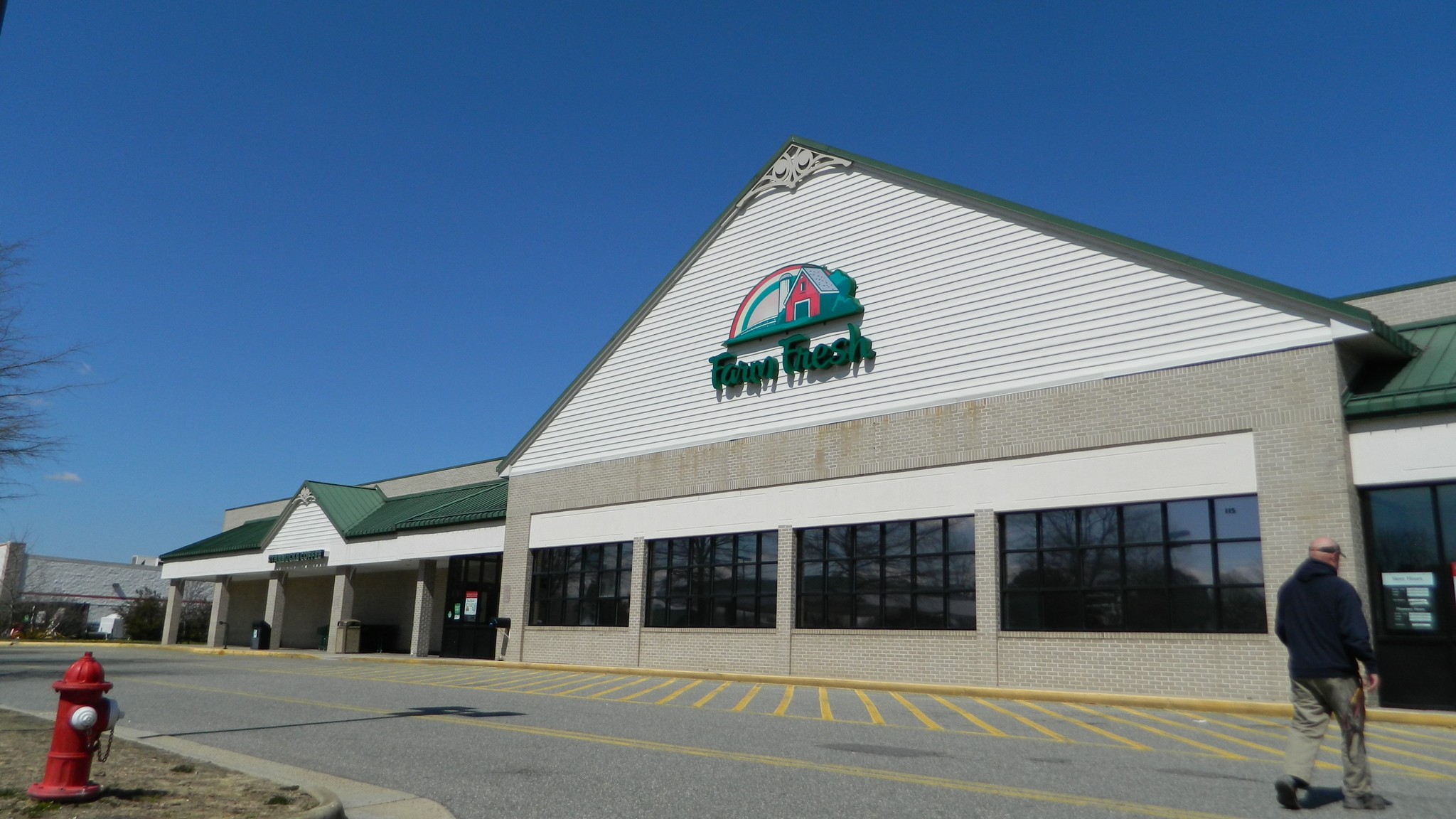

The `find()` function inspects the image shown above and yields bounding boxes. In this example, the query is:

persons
[1274,537,1385,810]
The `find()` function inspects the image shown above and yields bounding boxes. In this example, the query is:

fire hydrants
[25,649,125,805]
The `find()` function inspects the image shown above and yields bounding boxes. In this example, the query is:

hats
[1309,545,1347,559]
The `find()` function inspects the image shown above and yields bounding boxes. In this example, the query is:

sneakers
[1276,774,1302,809]
[1343,793,1386,809]
